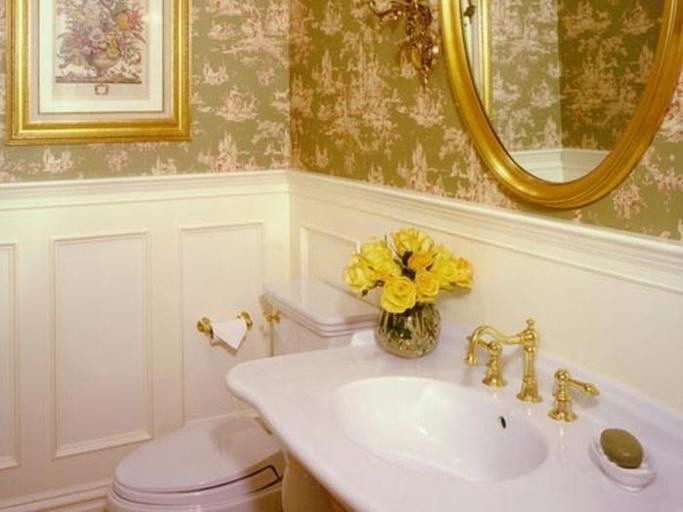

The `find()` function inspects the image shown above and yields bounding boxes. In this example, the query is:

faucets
[463,318,542,404]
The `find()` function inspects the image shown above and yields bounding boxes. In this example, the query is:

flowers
[341,227,473,316]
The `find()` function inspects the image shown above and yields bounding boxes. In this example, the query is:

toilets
[106,274,382,512]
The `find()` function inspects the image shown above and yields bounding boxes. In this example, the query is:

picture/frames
[6,0,191,146]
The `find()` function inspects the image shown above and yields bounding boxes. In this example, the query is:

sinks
[329,376,552,482]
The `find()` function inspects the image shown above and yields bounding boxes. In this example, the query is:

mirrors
[437,0,683,210]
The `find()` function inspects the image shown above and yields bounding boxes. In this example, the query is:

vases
[375,303,442,359]
